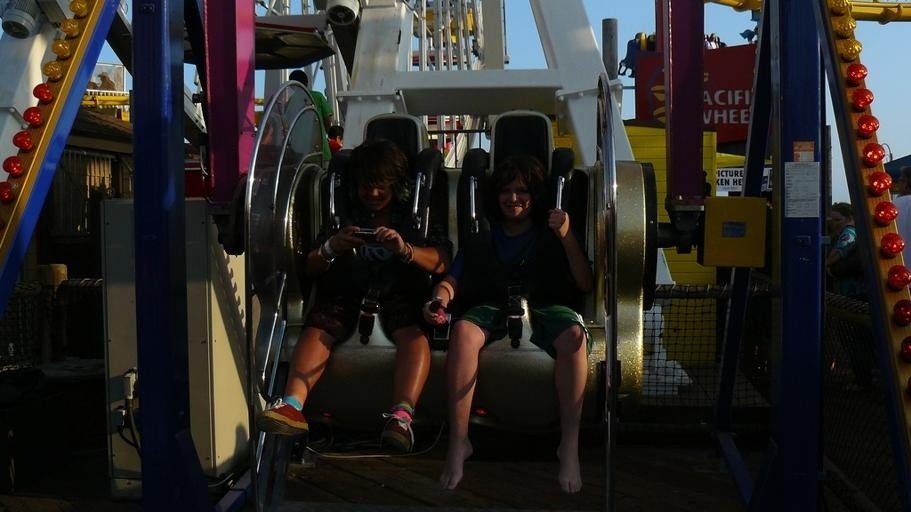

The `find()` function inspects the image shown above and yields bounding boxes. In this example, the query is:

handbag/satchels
[834,240,868,276]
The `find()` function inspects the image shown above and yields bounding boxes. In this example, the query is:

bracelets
[398,240,416,268]
[318,235,342,266]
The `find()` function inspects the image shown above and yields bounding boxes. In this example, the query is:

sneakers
[380,410,415,453]
[256,396,310,437]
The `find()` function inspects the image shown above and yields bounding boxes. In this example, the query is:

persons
[890,165,911,275]
[97,71,117,92]
[705,32,719,50]
[420,151,594,495]
[283,69,333,170]
[826,202,866,294]
[254,135,449,454]
[328,125,344,151]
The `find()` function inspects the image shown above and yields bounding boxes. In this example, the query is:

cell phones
[355,232,383,246]
